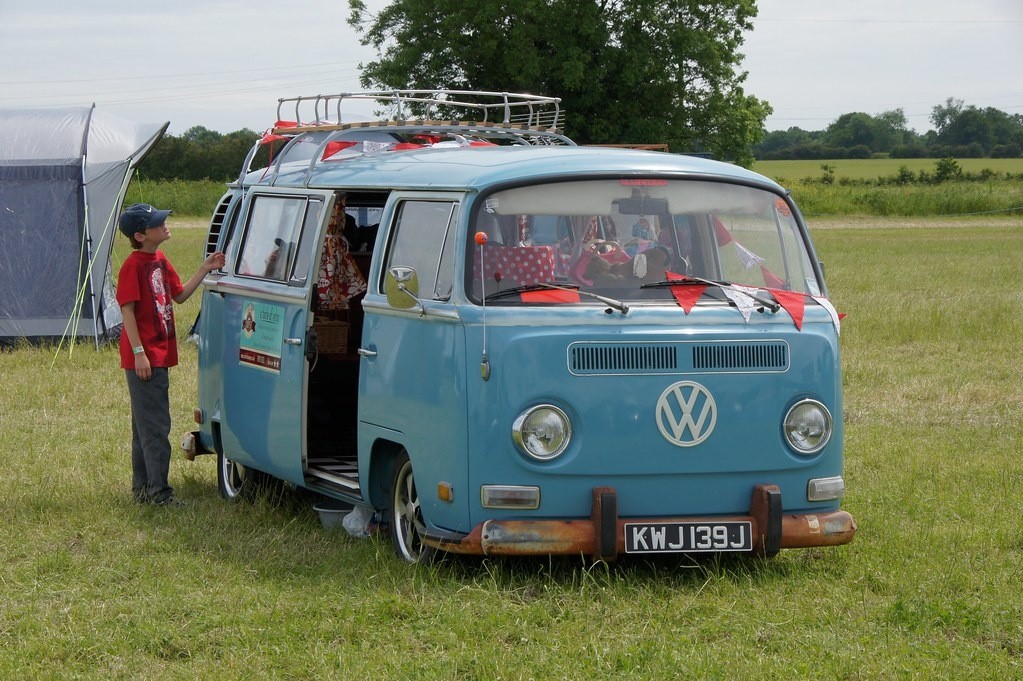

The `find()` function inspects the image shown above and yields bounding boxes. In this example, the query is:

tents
[1,104,170,373]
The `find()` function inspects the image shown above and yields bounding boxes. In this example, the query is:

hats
[119,203,173,238]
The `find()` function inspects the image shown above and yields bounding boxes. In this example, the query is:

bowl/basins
[312,504,353,530]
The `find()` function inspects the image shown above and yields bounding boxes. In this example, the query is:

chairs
[474,246,556,300]
[568,239,628,287]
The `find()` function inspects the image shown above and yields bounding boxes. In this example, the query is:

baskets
[308,316,350,354]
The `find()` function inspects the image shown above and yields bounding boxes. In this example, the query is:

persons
[114,205,226,504]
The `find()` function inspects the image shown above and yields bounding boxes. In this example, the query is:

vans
[181,89,856,572]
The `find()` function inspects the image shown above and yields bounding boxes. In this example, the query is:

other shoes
[133,494,151,507]
[151,495,184,507]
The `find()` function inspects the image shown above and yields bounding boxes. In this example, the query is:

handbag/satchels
[569,238,631,288]
